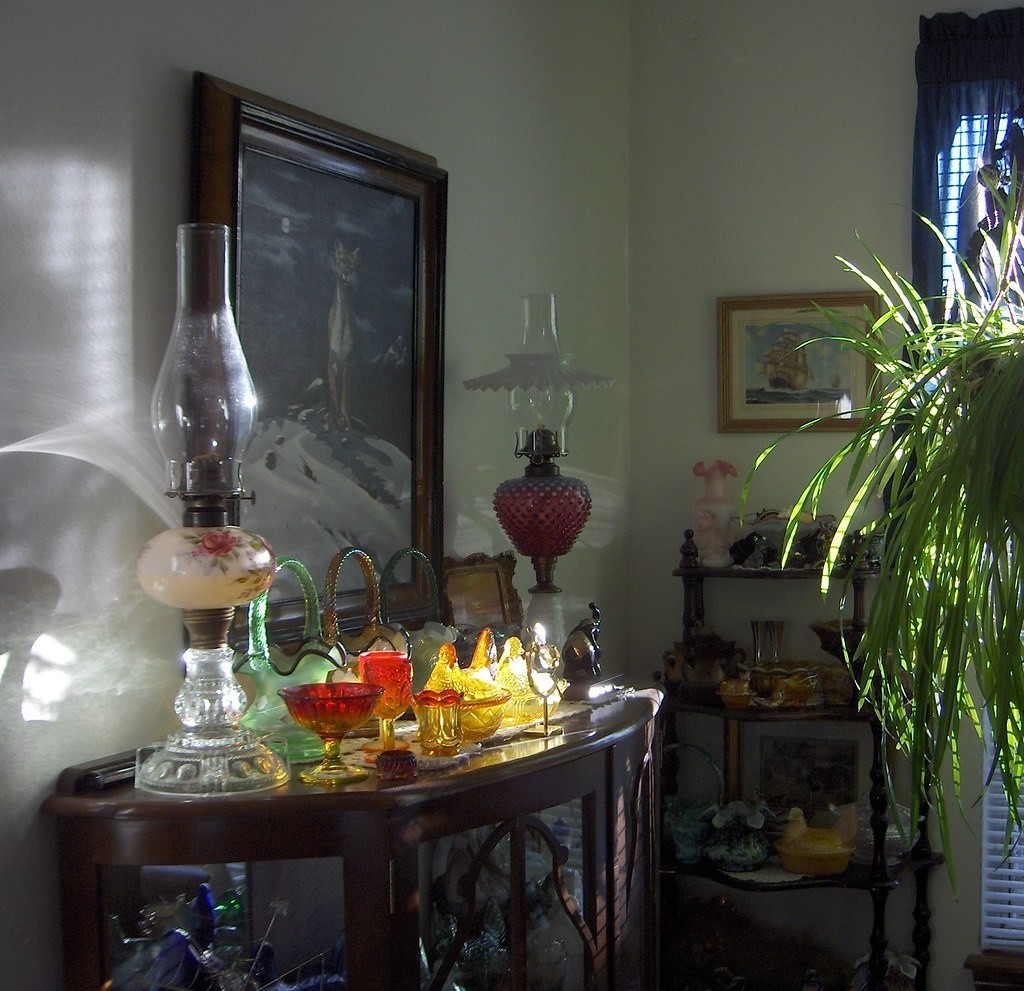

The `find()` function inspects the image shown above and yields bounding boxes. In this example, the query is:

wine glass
[359,651,412,752]
[276,680,386,786]
[366,656,413,764]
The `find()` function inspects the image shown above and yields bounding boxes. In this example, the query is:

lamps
[137,219,292,797]
[461,290,616,683]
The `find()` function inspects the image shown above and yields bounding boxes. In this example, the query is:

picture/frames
[717,291,880,432]
[760,735,859,811]
[182,70,449,680]
[427,553,525,666]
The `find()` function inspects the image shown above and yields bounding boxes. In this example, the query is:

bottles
[750,619,783,663]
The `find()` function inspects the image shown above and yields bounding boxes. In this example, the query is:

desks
[38,676,669,991]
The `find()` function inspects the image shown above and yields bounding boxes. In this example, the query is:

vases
[749,619,785,671]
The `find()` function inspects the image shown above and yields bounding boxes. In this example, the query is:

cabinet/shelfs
[648,526,945,991]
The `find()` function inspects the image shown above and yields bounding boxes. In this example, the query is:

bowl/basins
[415,689,511,744]
[505,678,569,723]
[716,689,755,708]
[749,664,819,696]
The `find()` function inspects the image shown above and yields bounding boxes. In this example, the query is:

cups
[413,689,464,756]
